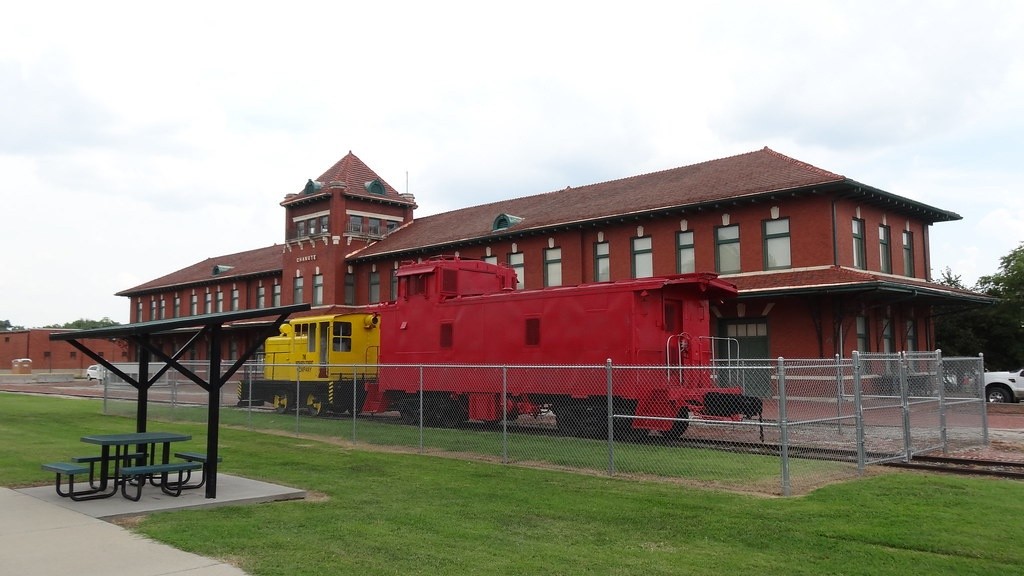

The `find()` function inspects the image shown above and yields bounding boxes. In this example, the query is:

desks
[80,432,193,497]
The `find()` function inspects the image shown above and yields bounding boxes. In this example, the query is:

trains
[262,255,763,442]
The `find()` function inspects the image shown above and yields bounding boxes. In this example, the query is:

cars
[86,365,105,381]
[984,369,1024,403]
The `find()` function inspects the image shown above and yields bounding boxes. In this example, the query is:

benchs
[168,453,222,490]
[120,462,202,502]
[42,462,107,501]
[72,452,149,489]
